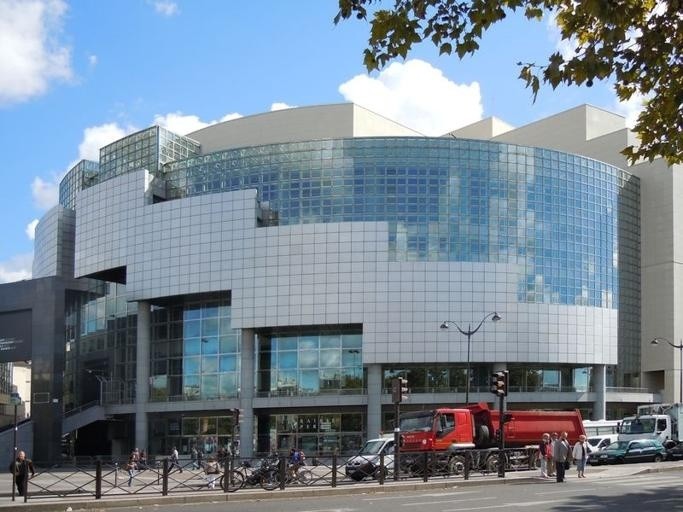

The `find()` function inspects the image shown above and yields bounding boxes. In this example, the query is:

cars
[587,436,683,465]
[345,438,395,479]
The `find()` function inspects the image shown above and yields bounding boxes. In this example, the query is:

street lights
[650,337,683,403]
[9,392,20,501]
[440,311,502,403]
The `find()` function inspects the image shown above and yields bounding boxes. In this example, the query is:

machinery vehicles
[400,401,587,477]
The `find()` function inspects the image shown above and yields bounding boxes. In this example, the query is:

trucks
[617,403,683,441]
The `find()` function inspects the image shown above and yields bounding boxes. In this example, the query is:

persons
[570,435,591,478]
[538,432,550,478]
[545,431,559,477]
[553,430,571,483]
[122,444,324,492]
[560,430,572,478]
[7,449,35,496]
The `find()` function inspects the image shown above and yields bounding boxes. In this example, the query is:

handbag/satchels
[587,446,593,453]
[565,461,572,469]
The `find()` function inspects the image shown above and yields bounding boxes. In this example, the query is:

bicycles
[219,460,314,492]
